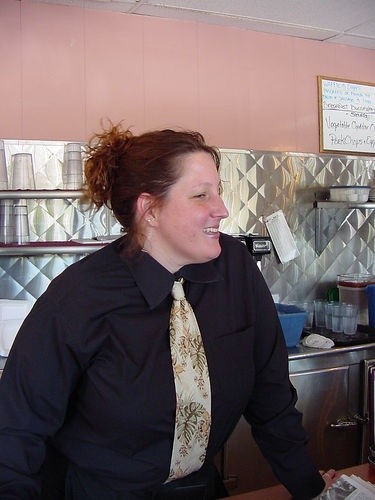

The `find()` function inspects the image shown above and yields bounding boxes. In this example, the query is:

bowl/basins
[329,185,371,204]
[275,303,310,348]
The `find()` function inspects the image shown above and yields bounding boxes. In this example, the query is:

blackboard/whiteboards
[318,75,375,155]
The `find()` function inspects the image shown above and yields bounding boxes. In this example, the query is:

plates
[369,195,375,202]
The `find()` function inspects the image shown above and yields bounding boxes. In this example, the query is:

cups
[64,142,85,190]
[0,198,30,247]
[299,299,358,335]
[11,153,36,190]
[1,140,9,190]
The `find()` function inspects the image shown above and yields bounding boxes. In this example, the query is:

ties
[161,278,214,484]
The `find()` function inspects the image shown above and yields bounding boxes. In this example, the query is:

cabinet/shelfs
[0,189,110,255]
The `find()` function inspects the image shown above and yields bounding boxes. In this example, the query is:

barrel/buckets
[336,273,375,336]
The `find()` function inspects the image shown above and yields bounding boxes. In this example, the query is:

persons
[0,125,336,500]
